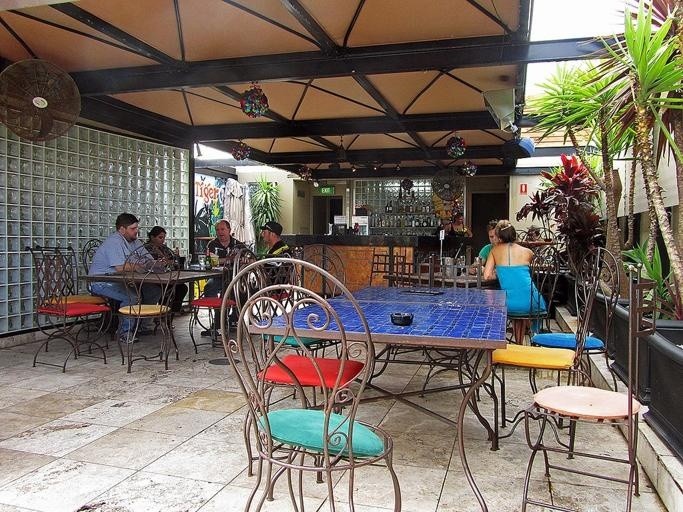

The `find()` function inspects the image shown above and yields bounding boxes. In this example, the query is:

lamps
[462,155,477,179]
[232,138,250,162]
[240,82,269,118]
[445,130,466,159]
[336,132,349,162]
[297,160,413,193]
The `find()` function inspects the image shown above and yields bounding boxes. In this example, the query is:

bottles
[172,248,180,271]
[448,220,454,234]
[372,213,441,227]
[354,223,359,234]
[386,191,431,213]
[205,248,212,269]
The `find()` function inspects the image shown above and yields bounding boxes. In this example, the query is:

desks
[233,284,508,462]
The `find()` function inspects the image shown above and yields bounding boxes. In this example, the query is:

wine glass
[211,256,220,271]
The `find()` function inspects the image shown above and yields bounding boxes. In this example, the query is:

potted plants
[516,135,681,463]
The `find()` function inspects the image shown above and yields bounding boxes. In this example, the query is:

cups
[182,253,192,269]
[445,255,483,277]
[197,255,205,271]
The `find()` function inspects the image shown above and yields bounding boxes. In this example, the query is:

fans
[0,58,80,142]
[430,167,462,201]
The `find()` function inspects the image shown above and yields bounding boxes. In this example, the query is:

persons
[484,220,547,344]
[140,226,188,317]
[443,214,473,238]
[468,221,496,274]
[229,221,293,327]
[88,212,167,344]
[522,227,546,251]
[201,220,256,336]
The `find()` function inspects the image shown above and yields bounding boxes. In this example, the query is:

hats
[260,221,283,235]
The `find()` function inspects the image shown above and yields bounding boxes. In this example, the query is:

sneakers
[201,328,222,337]
[115,329,139,344]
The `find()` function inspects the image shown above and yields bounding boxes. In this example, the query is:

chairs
[230,247,364,484]
[293,244,347,394]
[419,256,486,402]
[529,245,620,426]
[488,259,598,461]
[219,257,403,512]
[24,237,305,373]
[367,249,557,346]
[517,259,659,512]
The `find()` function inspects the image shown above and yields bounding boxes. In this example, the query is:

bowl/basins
[391,312,413,326]
